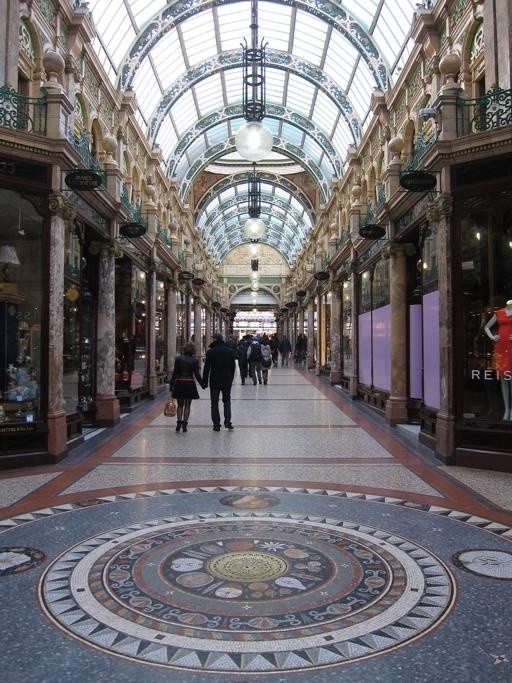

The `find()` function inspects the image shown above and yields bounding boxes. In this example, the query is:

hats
[211,333,223,340]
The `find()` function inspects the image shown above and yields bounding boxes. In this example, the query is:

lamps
[234,0,274,291]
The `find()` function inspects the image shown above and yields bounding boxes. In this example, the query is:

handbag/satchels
[263,358,271,368]
[164,402,176,416]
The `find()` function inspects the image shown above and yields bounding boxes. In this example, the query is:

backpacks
[252,344,260,361]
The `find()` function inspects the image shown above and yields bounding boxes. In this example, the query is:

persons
[169,342,205,431]
[484,300,512,421]
[202,333,235,431]
[226,332,307,385]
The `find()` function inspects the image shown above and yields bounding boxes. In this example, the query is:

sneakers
[213,427,220,431]
[225,423,234,429]
[241,378,267,386]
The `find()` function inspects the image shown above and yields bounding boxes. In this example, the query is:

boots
[176,421,182,432]
[183,422,188,432]
